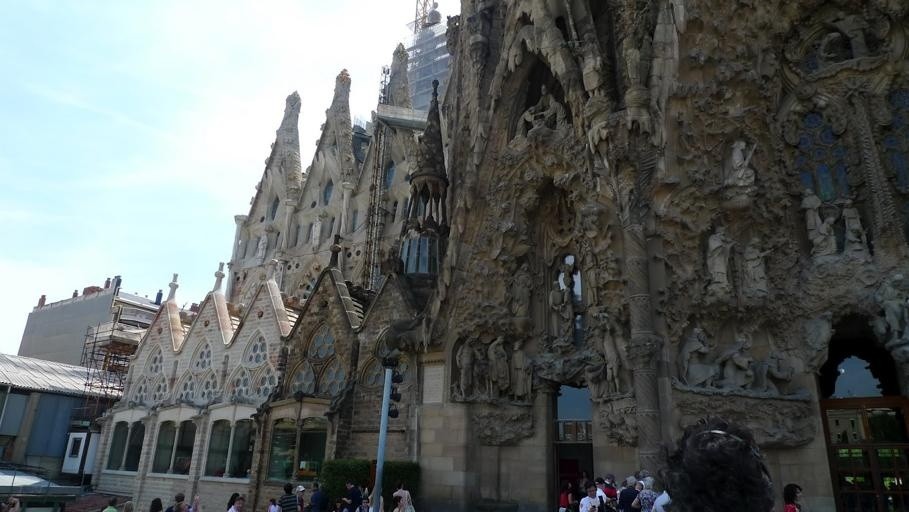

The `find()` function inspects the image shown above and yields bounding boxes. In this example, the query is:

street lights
[373,358,403,512]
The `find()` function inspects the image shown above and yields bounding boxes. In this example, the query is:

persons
[707,225,738,285]
[227,477,418,512]
[513,261,535,318]
[0,496,21,512]
[550,279,562,341]
[782,482,804,511]
[558,469,674,512]
[455,337,485,400]
[821,12,873,60]
[104,495,134,512]
[515,106,536,139]
[488,335,509,400]
[534,84,556,117]
[744,237,774,297]
[724,139,756,188]
[804,311,837,377]
[809,216,837,257]
[680,328,721,390]
[581,33,606,101]
[544,98,568,132]
[841,198,866,252]
[148,493,201,511]
[798,188,823,245]
[714,333,756,392]
[873,274,909,341]
[620,23,644,89]
[511,338,533,406]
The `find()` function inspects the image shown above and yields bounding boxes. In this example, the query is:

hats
[296,485,305,492]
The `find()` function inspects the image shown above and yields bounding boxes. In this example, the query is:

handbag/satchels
[403,491,415,511]
